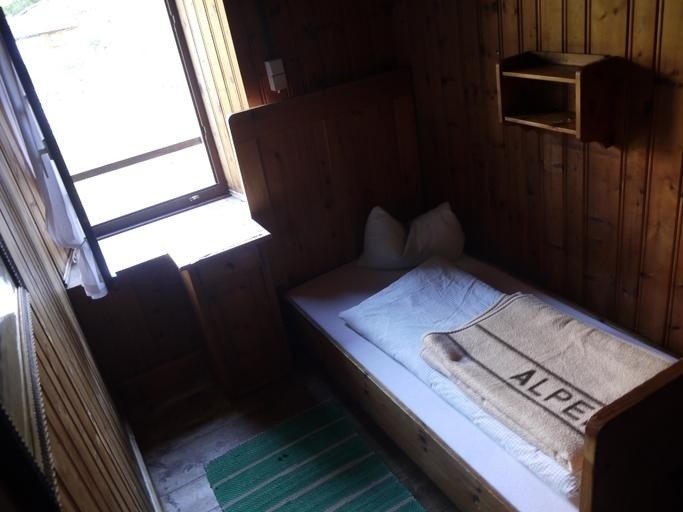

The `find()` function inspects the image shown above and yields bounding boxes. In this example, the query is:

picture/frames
[0,234,61,510]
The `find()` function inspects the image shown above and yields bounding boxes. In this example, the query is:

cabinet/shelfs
[494,49,615,142]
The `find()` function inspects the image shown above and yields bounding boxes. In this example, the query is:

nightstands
[167,208,278,372]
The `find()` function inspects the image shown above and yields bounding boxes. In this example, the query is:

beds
[229,67,681,510]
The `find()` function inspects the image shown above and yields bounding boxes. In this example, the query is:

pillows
[354,200,474,270]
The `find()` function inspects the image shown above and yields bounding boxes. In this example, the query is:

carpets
[202,397,423,509]
